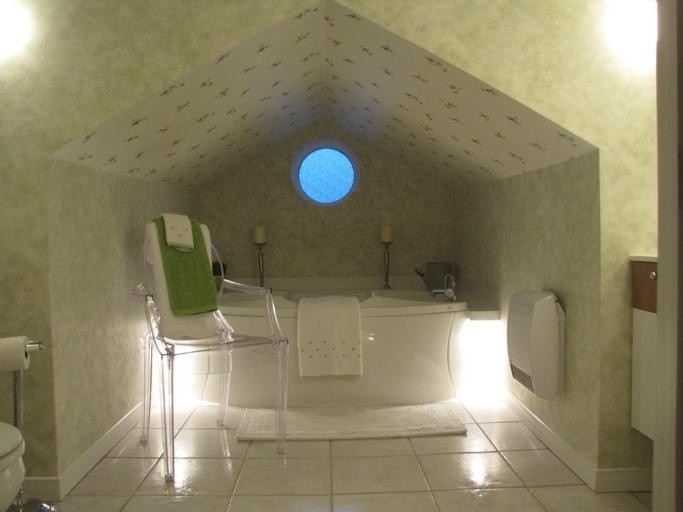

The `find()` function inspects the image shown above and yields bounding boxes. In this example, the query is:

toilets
[0,419,27,511]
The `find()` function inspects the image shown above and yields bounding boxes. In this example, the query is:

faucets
[430,275,457,302]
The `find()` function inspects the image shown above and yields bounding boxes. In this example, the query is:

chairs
[140,219,292,485]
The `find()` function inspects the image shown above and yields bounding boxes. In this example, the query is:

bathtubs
[188,287,466,409]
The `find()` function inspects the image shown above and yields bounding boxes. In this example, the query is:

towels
[152,211,219,318]
[297,296,364,378]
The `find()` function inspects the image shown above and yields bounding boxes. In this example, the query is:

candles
[253,226,265,243]
[382,224,393,242]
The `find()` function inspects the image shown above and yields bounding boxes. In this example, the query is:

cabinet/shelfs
[629,262,657,442]
